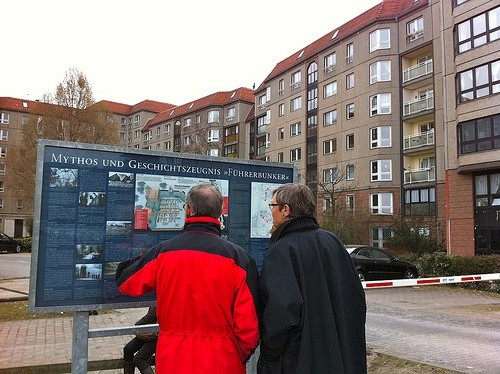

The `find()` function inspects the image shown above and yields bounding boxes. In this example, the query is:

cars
[0,231,22,253]
[344,245,419,281]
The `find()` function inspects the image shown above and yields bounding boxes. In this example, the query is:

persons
[123,303,158,374]
[116,183,262,374]
[257,183,367,374]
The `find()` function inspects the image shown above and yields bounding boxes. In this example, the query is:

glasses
[269,204,290,210]
[183,202,190,210]
